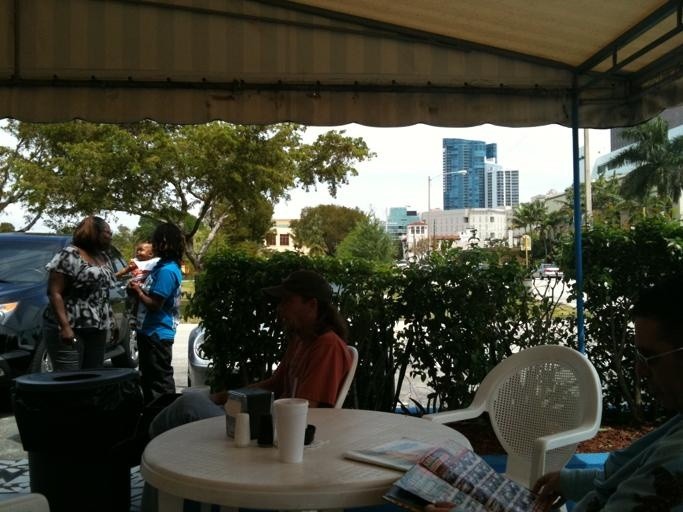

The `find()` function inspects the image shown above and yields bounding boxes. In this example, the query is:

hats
[261,270,333,304]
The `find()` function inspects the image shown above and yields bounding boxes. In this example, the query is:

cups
[233,413,250,448]
[273,399,309,463]
[305,425,316,445]
[257,414,273,448]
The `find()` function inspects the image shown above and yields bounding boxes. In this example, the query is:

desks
[141,405,473,512]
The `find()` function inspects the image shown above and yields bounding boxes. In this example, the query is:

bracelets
[137,291,143,298]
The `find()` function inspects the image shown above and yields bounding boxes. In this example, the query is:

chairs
[420,343,602,497]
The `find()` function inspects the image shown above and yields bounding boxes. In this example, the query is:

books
[380,435,562,511]
[340,432,441,471]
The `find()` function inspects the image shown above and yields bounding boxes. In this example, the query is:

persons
[139,268,352,512]
[41,215,131,371]
[421,275,683,511]
[113,242,163,331]
[126,222,186,412]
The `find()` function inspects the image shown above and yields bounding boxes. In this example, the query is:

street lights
[428,169,467,262]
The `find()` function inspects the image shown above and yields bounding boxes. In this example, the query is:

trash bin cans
[11,368,137,511]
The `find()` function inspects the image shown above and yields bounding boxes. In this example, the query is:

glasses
[633,347,683,368]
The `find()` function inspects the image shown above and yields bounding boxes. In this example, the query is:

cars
[0,230,139,395]
[538,263,563,280]
[187,281,395,412]
